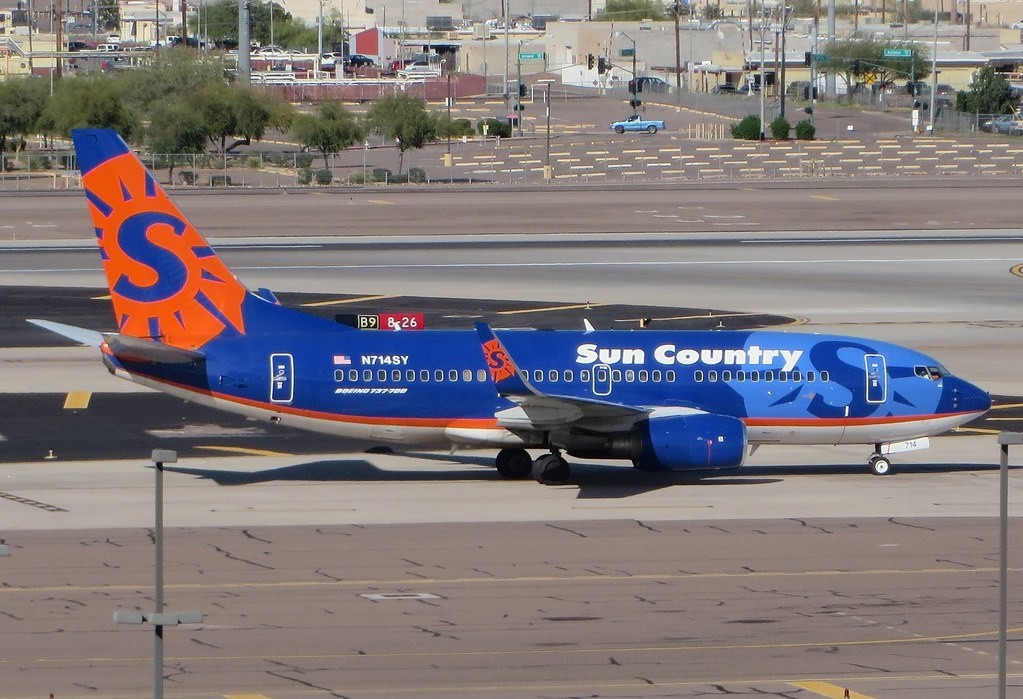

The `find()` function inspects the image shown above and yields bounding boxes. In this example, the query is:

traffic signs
[519,52,544,59]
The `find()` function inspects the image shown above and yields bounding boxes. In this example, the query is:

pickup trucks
[612,115,665,134]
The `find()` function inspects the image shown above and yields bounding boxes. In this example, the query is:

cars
[340,55,374,67]
[121,36,207,52]
[712,84,738,95]
[1011,16,1023,29]
[391,59,414,72]
[739,86,760,96]
[785,80,810,98]
[983,113,1023,133]
[249,46,302,54]
[320,52,343,63]
[404,61,431,71]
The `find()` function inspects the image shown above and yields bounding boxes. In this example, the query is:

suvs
[634,76,672,94]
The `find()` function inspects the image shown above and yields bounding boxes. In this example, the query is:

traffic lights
[805,52,812,68]
[637,81,643,93]
[588,53,595,69]
[520,84,527,97]
[628,81,635,92]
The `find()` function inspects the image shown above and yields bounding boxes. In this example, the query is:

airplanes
[24,126,996,487]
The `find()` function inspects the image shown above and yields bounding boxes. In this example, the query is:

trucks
[78,43,119,52]
